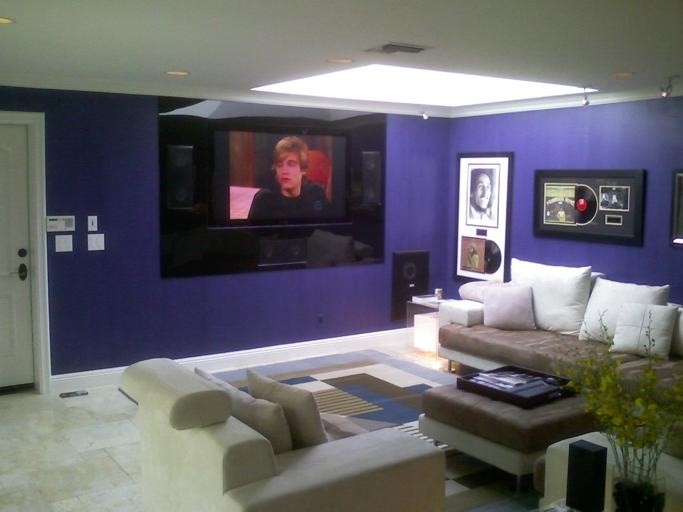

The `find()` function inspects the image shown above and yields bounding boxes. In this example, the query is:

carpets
[212,347,533,512]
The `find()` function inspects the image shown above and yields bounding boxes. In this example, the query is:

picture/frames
[668,169,683,247]
[534,169,647,246]
[452,151,515,284]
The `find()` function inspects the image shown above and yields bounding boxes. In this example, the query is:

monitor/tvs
[211,131,352,226]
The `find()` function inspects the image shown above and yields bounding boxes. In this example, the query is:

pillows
[590,270,606,292]
[510,258,592,337]
[667,303,682,355]
[484,286,535,330]
[578,276,670,345]
[247,368,328,448]
[607,302,679,361]
[195,368,292,454]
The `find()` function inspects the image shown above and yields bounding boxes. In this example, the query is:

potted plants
[552,309,682,511]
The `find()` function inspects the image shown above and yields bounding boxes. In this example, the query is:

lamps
[582,87,590,106]
[660,78,674,98]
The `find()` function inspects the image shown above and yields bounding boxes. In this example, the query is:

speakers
[361,151,383,209]
[168,143,195,211]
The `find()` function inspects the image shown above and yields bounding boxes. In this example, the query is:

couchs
[414,272,683,413]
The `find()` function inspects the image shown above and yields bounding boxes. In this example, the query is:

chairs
[118,357,446,511]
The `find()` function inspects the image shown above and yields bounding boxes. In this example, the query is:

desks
[534,498,616,512]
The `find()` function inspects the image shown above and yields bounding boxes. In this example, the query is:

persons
[248,136,329,216]
[470,168,493,221]
[465,244,480,269]
